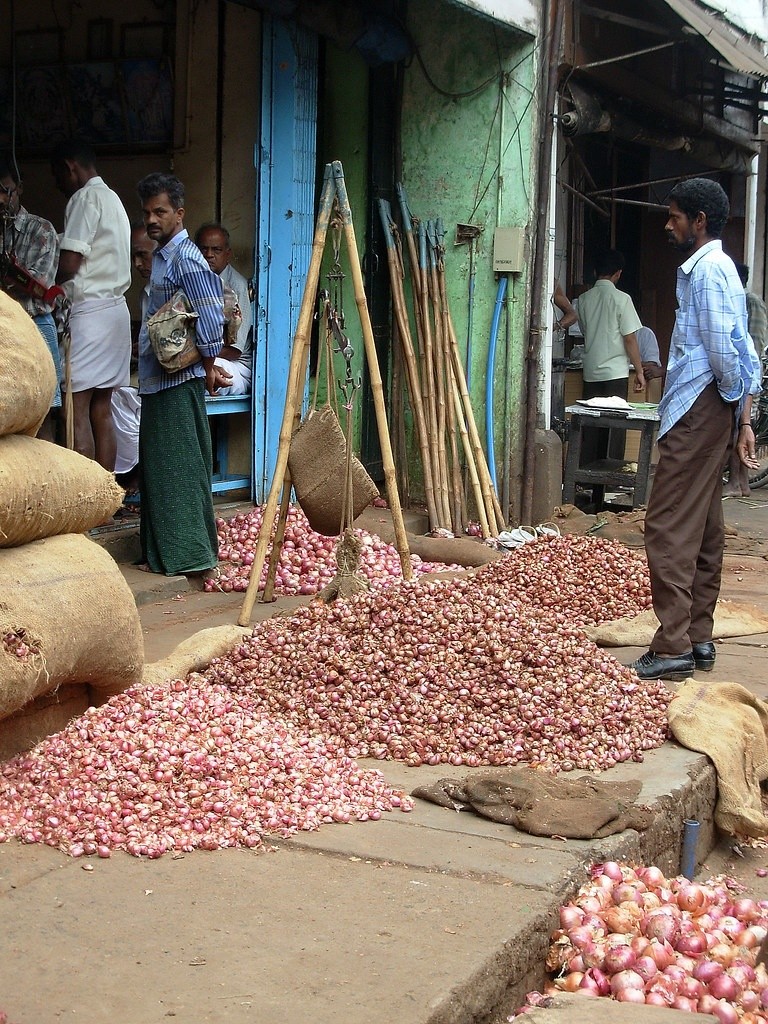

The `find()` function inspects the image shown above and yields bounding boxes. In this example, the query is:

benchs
[125,394,253,503]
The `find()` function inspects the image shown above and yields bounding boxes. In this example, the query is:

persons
[190,222,254,398]
[138,172,235,577]
[0,161,62,444]
[621,176,762,681]
[552,252,768,498]
[49,145,133,472]
[129,223,159,317]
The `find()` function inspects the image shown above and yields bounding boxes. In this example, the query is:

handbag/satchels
[145,285,242,373]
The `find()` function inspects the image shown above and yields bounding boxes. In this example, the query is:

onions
[0,496,684,861]
[502,861,768,1024]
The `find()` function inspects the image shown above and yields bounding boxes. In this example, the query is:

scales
[314,247,363,389]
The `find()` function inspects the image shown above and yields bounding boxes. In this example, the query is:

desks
[565,398,662,509]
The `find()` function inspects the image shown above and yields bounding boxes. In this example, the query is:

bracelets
[739,423,752,427]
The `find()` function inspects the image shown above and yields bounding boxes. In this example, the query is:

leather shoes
[692,642,716,670]
[622,647,695,682]
